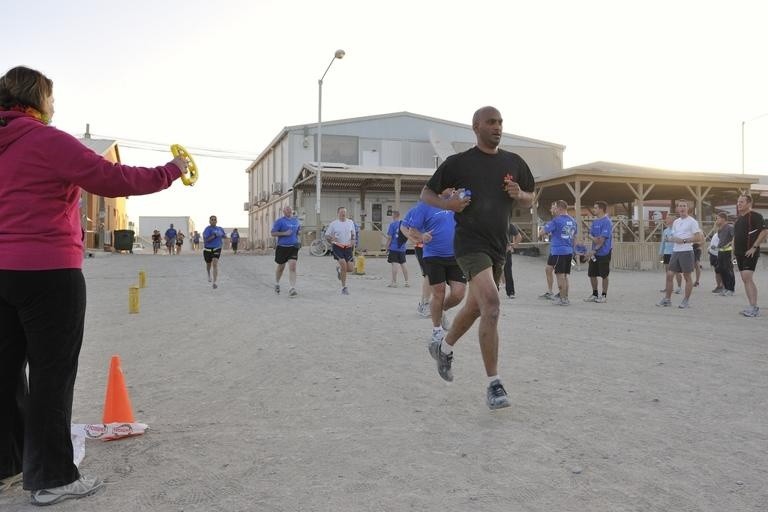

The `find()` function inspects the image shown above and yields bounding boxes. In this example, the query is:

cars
[131,243,143,255]
[160,240,177,254]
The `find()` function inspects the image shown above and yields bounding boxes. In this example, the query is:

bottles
[591,255,596,262]
[460,190,471,200]
[544,234,549,241]
[571,258,576,266]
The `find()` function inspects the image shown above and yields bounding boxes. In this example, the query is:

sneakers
[275,284,280,293]
[290,288,297,297]
[486,379,511,410]
[336,264,342,280]
[342,287,349,294]
[30,472,104,506]
[595,295,606,302]
[539,292,569,304]
[584,295,597,302]
[386,281,397,288]
[416,301,451,341]
[428,337,455,381]
[0,471,24,490]
[740,306,759,317]
[404,280,410,287]
[656,282,736,308]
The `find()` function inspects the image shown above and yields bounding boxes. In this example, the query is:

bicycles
[310,226,330,257]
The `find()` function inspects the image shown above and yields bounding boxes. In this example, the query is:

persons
[420,104,535,411]
[657,194,766,316]
[0,66,189,507]
[154,215,240,288]
[153,190,767,347]
[539,200,613,305]
[326,206,356,293]
[401,202,522,343]
[384,211,410,287]
[271,208,302,295]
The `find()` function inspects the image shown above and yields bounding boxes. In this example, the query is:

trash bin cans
[114,230,134,253]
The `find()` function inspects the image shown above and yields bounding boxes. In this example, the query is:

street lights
[316,46,344,239]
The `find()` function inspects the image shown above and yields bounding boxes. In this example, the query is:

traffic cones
[99,353,143,443]
